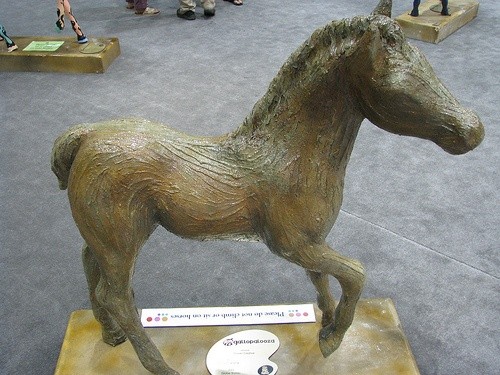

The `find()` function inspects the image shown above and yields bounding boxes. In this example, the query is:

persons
[126,0,160,16]
[177,0,216,20]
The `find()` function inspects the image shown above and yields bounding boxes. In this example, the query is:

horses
[50,0,487,375]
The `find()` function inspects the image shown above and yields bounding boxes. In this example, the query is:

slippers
[134,7,160,16]
[126,3,148,10]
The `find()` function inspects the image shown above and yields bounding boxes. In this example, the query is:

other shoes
[204,8,215,18]
[177,7,197,20]
[223,0,243,6]
